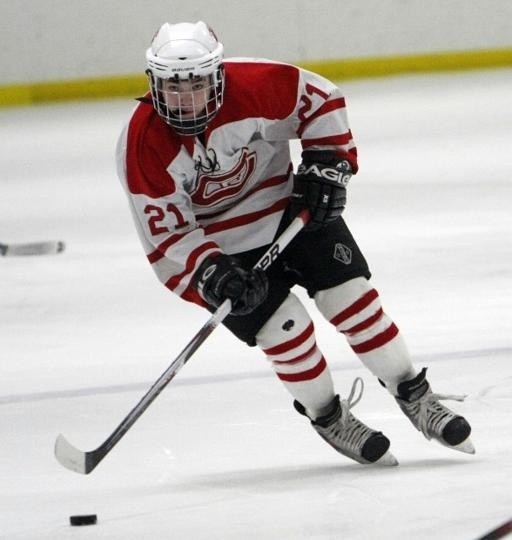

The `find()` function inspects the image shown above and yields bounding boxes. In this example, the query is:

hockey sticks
[0,241,62,256]
[54,202,309,474]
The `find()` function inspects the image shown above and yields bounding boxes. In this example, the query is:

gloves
[191,253,269,316]
[289,149,352,232]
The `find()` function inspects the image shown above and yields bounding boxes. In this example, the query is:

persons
[109,17,477,466]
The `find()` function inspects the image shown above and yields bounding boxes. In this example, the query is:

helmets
[145,19,225,136]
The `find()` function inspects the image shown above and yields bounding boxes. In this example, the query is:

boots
[294,393,390,465]
[378,367,471,446]
